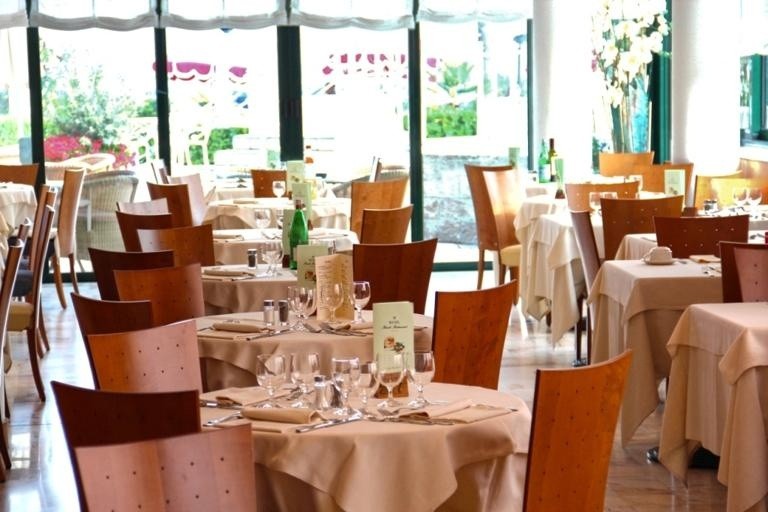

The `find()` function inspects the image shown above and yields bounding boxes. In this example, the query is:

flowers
[591,3,671,110]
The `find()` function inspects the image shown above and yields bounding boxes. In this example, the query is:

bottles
[247,249,258,268]
[705,199,718,213]
[264,299,287,327]
[539,137,557,183]
[289,198,308,269]
[303,144,316,198]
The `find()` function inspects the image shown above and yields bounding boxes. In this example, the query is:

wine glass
[734,188,763,218]
[288,282,371,331]
[253,181,286,276]
[589,191,604,217]
[255,351,435,415]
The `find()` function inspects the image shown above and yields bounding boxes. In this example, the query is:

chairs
[0,149,768,511]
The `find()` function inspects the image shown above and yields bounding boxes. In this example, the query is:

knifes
[295,417,360,432]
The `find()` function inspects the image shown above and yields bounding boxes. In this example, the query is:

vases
[609,125,632,151]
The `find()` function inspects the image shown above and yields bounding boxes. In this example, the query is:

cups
[644,247,672,260]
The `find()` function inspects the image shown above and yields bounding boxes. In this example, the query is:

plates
[643,257,676,264]
